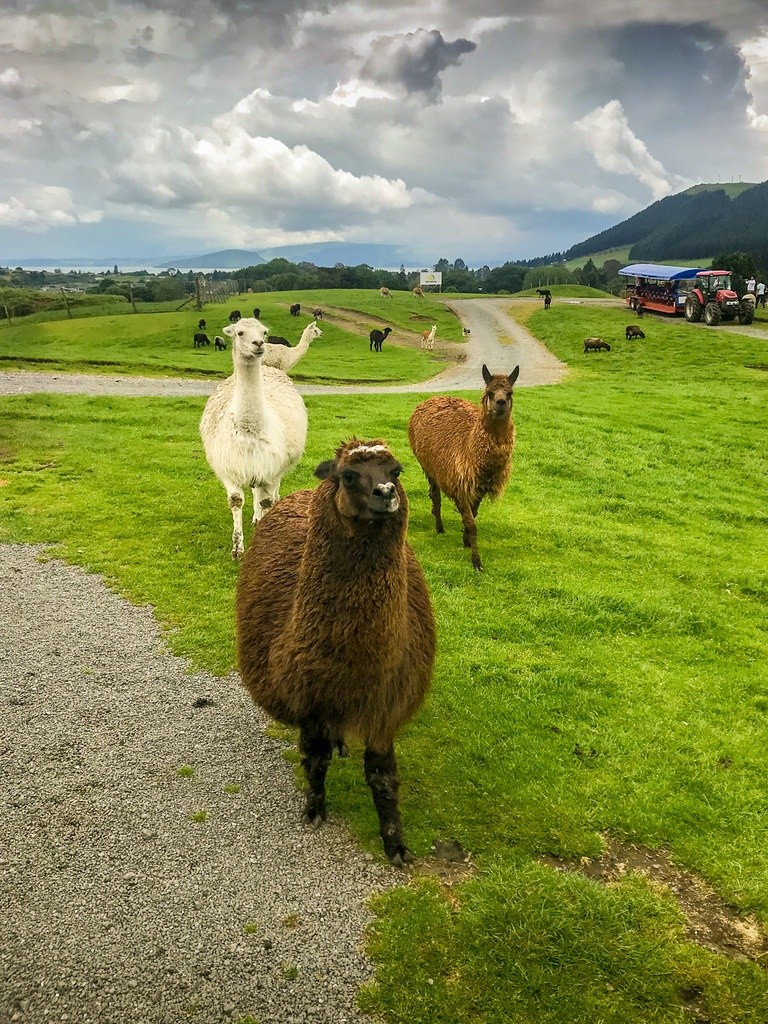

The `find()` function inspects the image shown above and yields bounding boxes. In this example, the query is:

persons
[745,277,756,293]
[713,279,721,287]
[755,281,766,309]
[672,281,680,289]
[636,278,642,286]
[658,283,673,294]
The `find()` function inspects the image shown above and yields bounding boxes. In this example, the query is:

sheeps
[193,333,210,349]
[312,308,322,320]
[535,288,552,299]
[584,337,611,353]
[420,324,437,350]
[638,303,646,318]
[545,295,551,311]
[235,435,440,871]
[198,318,206,330]
[195,316,307,568]
[229,310,241,323]
[253,307,260,320]
[369,325,392,352]
[411,362,526,576]
[412,287,425,297]
[289,303,301,316]
[214,335,226,352]
[268,335,292,347]
[379,287,393,297]
[625,325,645,341]
[261,319,324,378]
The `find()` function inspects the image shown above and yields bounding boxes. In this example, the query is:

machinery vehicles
[617,263,755,327]
